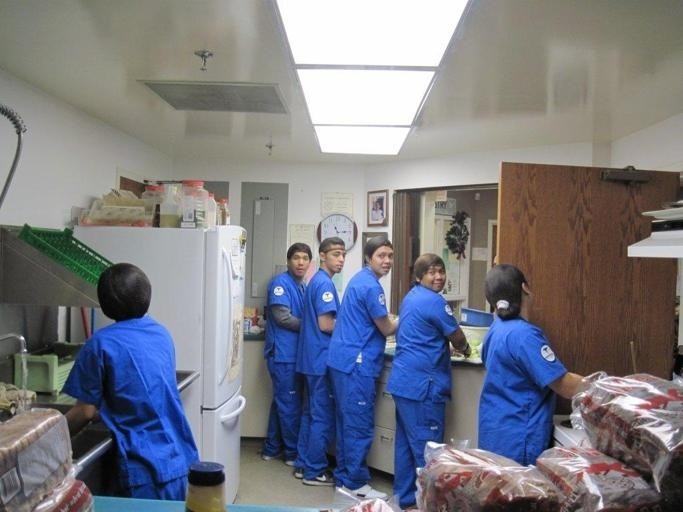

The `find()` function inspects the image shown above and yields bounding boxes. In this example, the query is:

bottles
[142,178,230,228]
[184,461,226,512]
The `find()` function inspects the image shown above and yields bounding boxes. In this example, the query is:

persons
[62,262,200,501]
[387,252,471,509]
[293,236,346,486]
[261,242,312,465]
[371,201,383,221]
[325,236,399,500]
[478,264,590,467]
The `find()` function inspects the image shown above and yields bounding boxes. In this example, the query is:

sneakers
[335,483,389,504]
[302,472,336,486]
[294,467,306,479]
[263,451,283,461]
[285,458,295,465]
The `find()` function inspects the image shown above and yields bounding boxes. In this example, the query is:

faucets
[0,333,28,353]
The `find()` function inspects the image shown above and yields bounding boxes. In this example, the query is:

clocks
[317,212,358,256]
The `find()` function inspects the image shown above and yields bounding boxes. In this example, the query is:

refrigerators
[67,225,247,506]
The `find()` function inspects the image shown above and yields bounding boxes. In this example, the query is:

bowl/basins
[460,306,493,327]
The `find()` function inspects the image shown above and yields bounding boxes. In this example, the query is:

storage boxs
[11,339,87,398]
[459,305,495,327]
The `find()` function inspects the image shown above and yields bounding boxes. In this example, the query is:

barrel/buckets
[182,179,209,229]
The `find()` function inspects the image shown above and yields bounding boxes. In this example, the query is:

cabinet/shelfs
[362,349,397,476]
[63,365,202,500]
[547,410,588,449]
[441,358,488,450]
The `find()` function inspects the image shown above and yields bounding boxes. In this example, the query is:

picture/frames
[361,231,390,270]
[365,188,390,229]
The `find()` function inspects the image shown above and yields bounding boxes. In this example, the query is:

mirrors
[389,181,504,329]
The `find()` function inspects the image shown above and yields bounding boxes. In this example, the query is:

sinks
[71,430,112,460]
